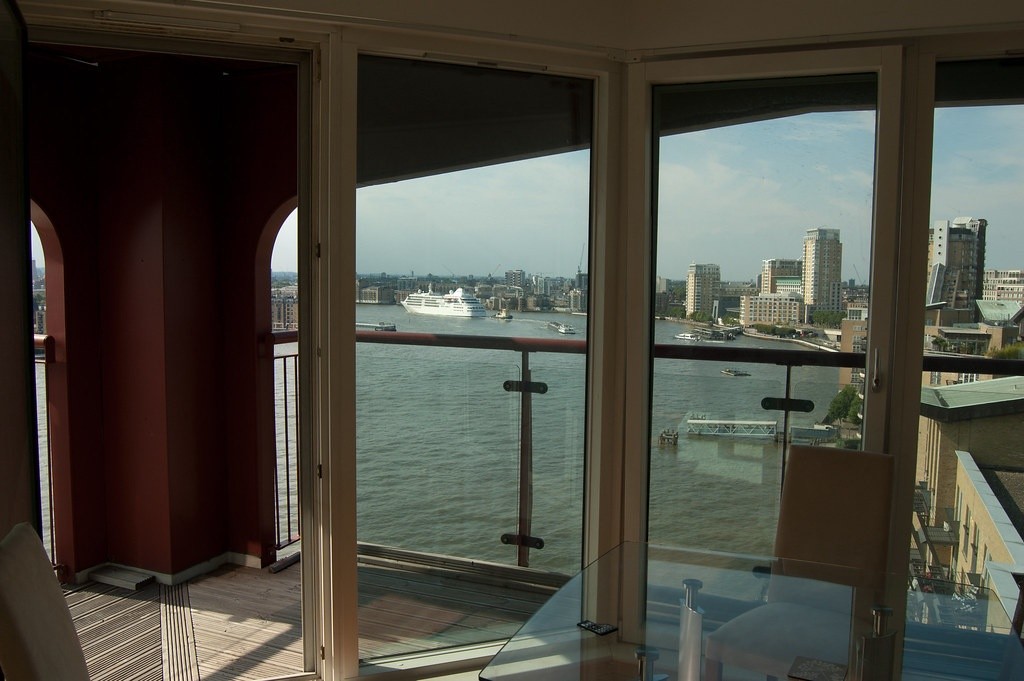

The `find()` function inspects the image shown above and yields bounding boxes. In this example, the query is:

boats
[675,333,701,342]
[548,321,575,336]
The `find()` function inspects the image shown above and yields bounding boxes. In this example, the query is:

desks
[476,540,1016,681]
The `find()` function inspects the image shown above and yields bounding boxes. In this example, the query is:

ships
[401,281,488,317]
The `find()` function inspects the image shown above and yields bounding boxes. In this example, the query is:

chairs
[704,444,892,681]
